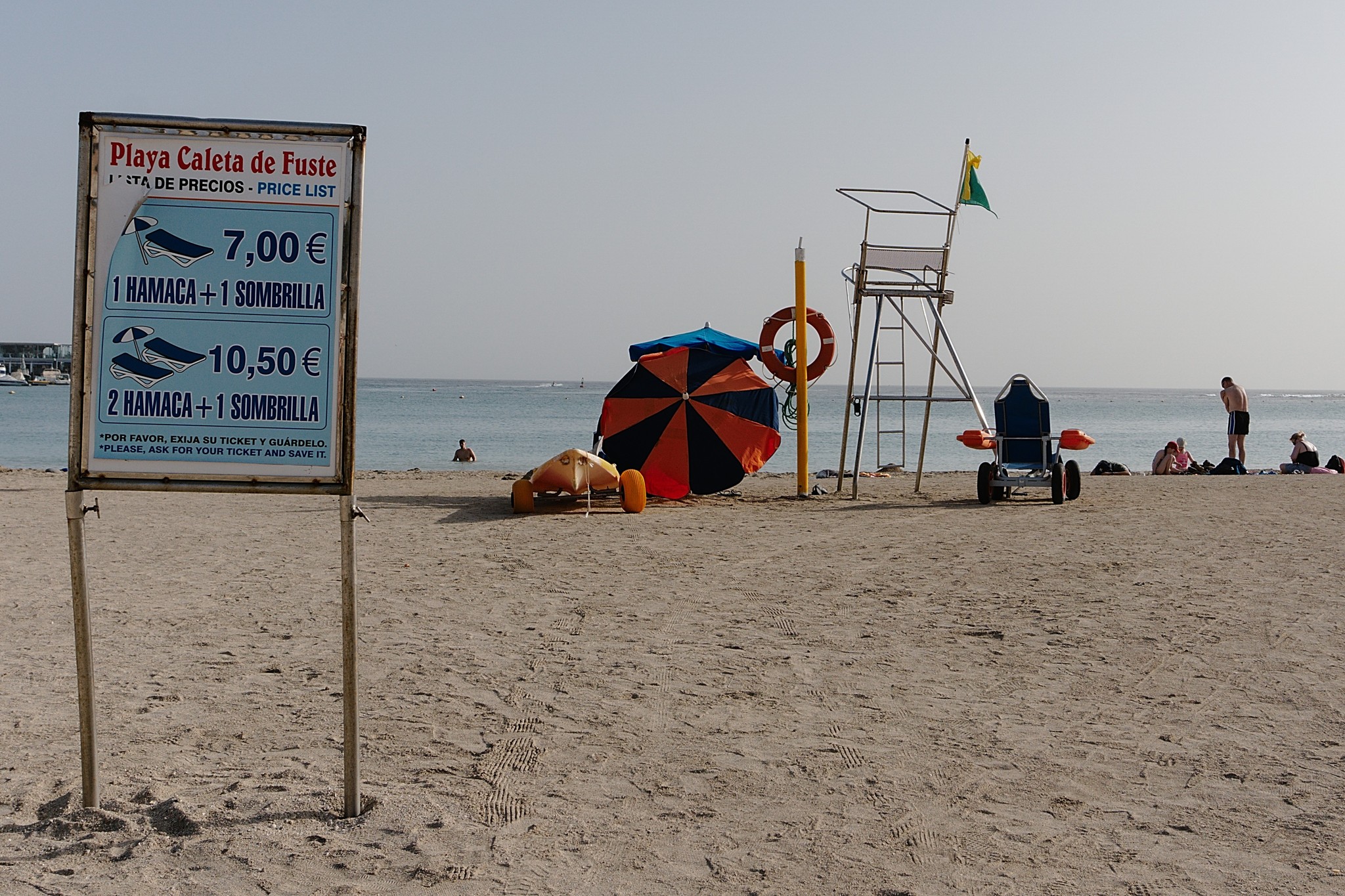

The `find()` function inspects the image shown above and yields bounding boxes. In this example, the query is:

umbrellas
[599,346,781,499]
[629,322,793,367]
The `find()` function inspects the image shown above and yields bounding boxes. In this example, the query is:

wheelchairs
[956,374,1095,504]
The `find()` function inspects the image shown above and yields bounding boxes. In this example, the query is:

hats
[1167,441,1180,453]
[1095,463,1110,475]
[1177,437,1187,447]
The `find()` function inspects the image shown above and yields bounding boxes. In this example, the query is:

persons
[1172,437,1195,470]
[1152,441,1183,475]
[1220,376,1250,465]
[1090,460,1131,475]
[1279,432,1320,474]
[453,439,476,461]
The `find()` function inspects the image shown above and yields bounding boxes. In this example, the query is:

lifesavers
[759,306,835,384]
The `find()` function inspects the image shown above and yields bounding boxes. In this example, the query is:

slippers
[1268,471,1277,474]
[1258,470,1268,475]
[1179,472,1185,475]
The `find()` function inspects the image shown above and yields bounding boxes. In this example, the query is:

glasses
[1222,381,1226,388]
[1293,438,1298,445]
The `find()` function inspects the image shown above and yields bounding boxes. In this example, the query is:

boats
[42,369,71,385]
[509,449,646,515]
[27,375,51,386]
[0,353,31,386]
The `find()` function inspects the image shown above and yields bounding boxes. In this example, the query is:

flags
[958,153,997,216]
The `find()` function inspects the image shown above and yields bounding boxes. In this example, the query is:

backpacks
[1325,455,1345,474]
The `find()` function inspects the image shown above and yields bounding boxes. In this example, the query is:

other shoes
[1210,457,1247,475]
